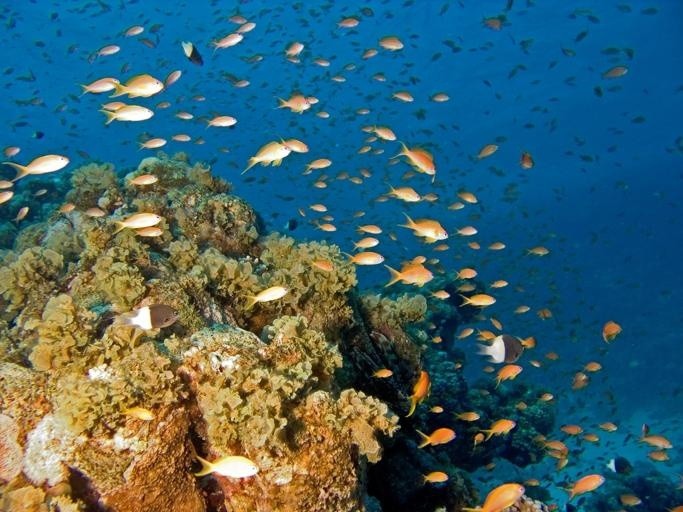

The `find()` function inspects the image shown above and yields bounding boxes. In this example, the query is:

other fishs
[1,3,681,512]
